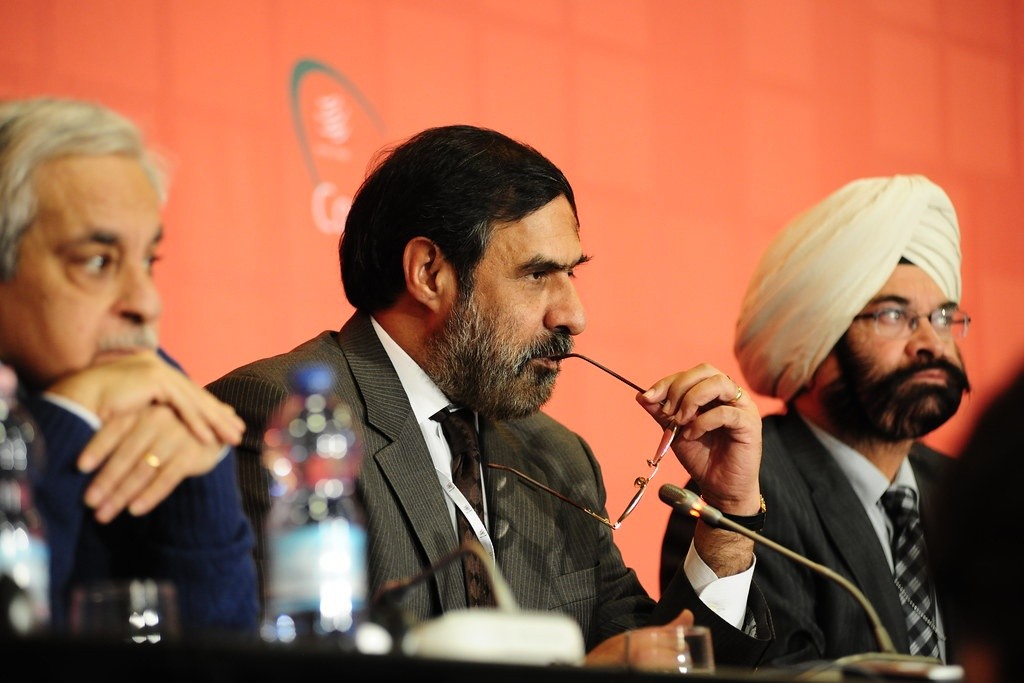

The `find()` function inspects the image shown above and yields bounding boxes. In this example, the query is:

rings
[728,385,742,403]
[146,453,160,470]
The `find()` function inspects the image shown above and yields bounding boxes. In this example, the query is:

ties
[876,484,943,658]
[430,407,498,608]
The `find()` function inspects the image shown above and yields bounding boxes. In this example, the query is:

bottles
[0,364,49,635]
[259,365,367,652]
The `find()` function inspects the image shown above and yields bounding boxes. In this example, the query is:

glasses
[854,302,971,342]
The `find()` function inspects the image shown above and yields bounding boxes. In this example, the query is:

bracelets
[699,494,766,534]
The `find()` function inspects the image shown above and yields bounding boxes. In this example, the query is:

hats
[733,176,962,401]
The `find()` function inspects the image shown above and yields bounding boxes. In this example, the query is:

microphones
[371,541,586,668]
[658,482,944,677]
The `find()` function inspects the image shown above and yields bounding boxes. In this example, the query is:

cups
[70,585,178,645]
[625,628,714,675]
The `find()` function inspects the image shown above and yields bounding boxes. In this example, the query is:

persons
[656,172,970,683]
[0,102,262,643]
[204,123,767,676]
